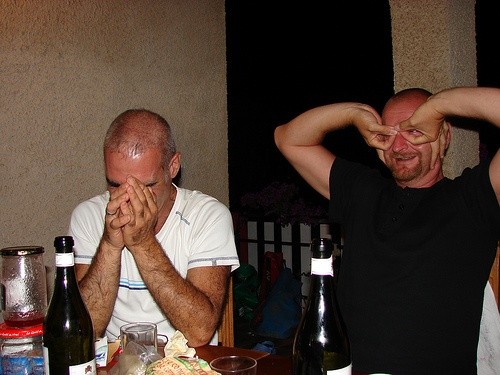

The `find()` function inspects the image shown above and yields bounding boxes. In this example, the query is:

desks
[156,344,368,375]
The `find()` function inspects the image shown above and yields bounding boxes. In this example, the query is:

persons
[68,108,240,348]
[274,86,500,375]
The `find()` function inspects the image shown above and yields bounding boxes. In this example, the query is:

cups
[119,322,158,354]
[155,333,169,359]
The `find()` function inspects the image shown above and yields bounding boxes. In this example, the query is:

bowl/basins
[209,356,258,375]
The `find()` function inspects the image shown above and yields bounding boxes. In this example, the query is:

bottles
[42,234,97,375]
[288,238,353,375]
[0,245,48,327]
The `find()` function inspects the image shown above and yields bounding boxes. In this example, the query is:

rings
[106,209,117,216]
